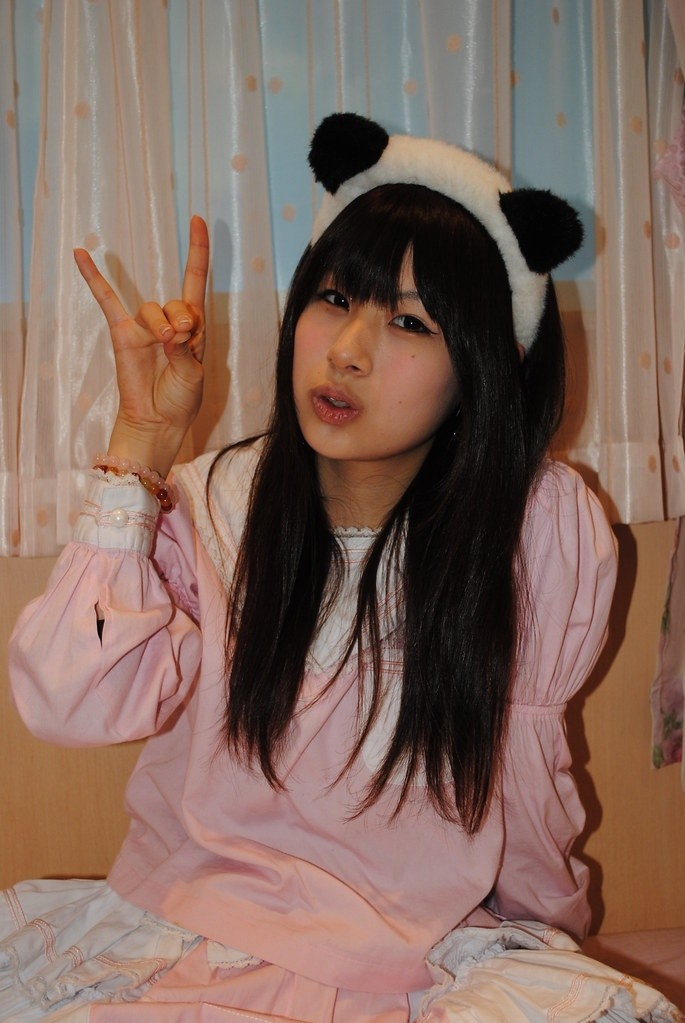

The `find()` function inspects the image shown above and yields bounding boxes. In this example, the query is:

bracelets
[93,453,176,514]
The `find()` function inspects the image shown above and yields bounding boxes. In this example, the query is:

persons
[0,112,685,1023]
